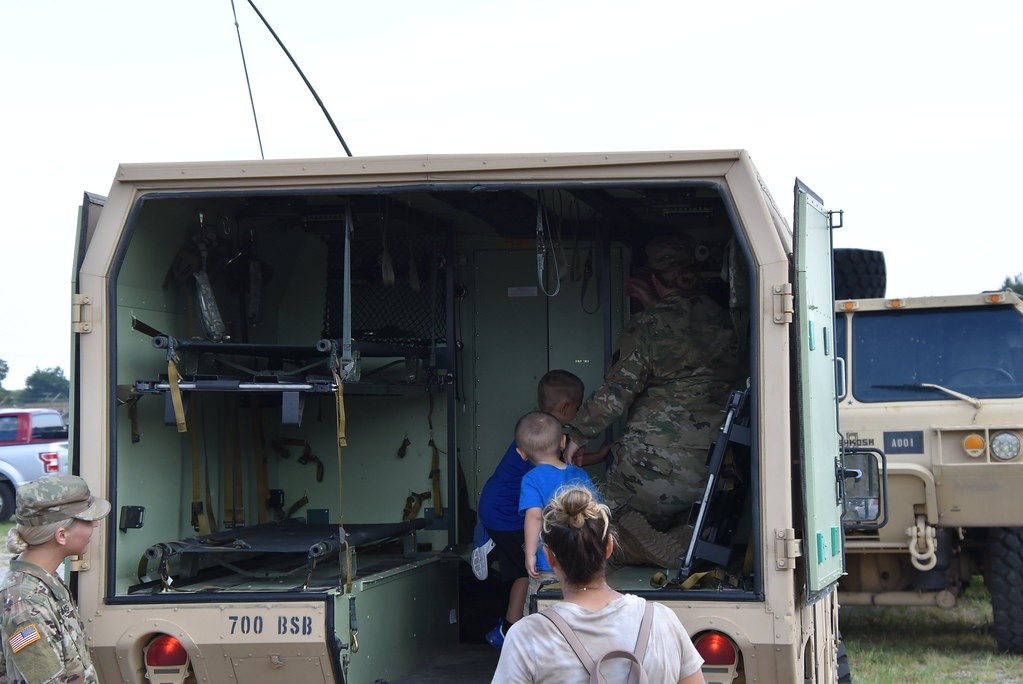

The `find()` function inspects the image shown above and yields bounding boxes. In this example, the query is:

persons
[515,410,605,649]
[562,231,745,570]
[470,370,613,648]
[490,489,705,684]
[0,475,111,684]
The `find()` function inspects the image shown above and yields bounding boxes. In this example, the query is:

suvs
[0,408,68,523]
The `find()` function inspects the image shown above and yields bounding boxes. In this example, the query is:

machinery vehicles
[64,148,888,684]
[834,248,1023,653]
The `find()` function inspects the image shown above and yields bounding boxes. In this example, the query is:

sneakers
[470,522,498,581]
[486,619,505,651]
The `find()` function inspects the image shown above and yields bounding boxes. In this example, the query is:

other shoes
[668,524,711,543]
[614,512,685,568]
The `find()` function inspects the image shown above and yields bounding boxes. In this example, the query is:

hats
[14,474,112,527]
[640,234,697,272]
